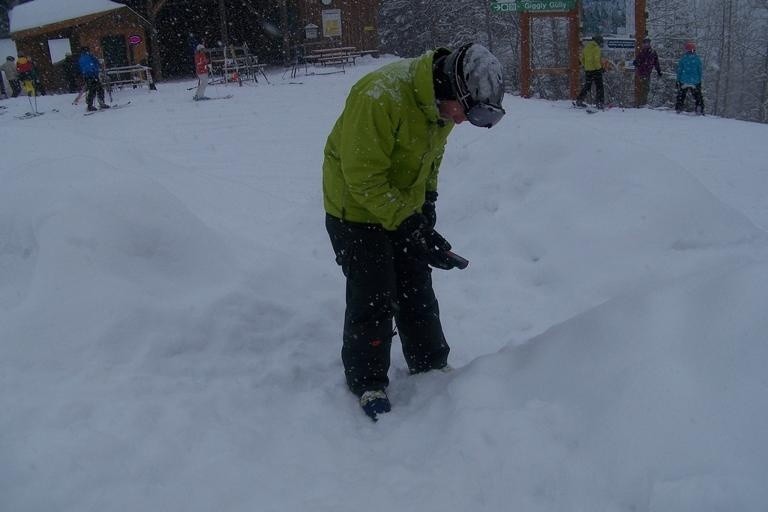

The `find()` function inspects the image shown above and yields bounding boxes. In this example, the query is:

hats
[196,44,205,51]
[444,43,505,109]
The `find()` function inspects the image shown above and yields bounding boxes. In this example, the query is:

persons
[675,41,705,116]
[194,44,209,100]
[633,39,663,108]
[576,35,605,109]
[79,46,111,111]
[0,50,47,97]
[323,43,506,423]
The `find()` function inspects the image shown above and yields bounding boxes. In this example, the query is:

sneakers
[88,104,110,111]
[195,95,211,99]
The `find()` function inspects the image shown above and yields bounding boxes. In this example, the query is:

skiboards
[13,109,60,119]
[144,53,158,91]
[191,95,232,100]
[71,82,86,106]
[243,42,270,85]
[223,44,242,85]
[84,101,131,116]
[568,105,612,112]
[672,111,709,117]
[100,48,115,103]
[625,105,654,109]
[129,45,138,89]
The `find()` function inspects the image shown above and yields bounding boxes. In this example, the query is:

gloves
[395,191,454,270]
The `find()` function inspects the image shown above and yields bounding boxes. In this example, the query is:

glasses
[466,103,506,128]
